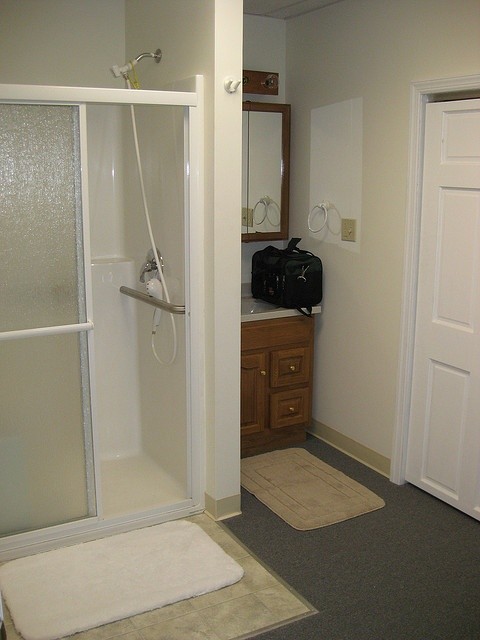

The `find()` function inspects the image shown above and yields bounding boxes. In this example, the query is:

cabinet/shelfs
[241,314,323,458]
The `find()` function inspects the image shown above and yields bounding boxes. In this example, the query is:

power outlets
[340,217,358,242]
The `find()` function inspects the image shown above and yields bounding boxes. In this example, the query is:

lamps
[260,74,279,90]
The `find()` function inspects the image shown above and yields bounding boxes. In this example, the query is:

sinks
[241,297,280,315]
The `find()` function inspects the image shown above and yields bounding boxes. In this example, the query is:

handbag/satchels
[251,238,323,319]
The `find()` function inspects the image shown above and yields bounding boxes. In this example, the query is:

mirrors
[243,102,290,241]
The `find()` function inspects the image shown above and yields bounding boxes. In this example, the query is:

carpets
[240,447,384,532]
[0,513,317,639]
